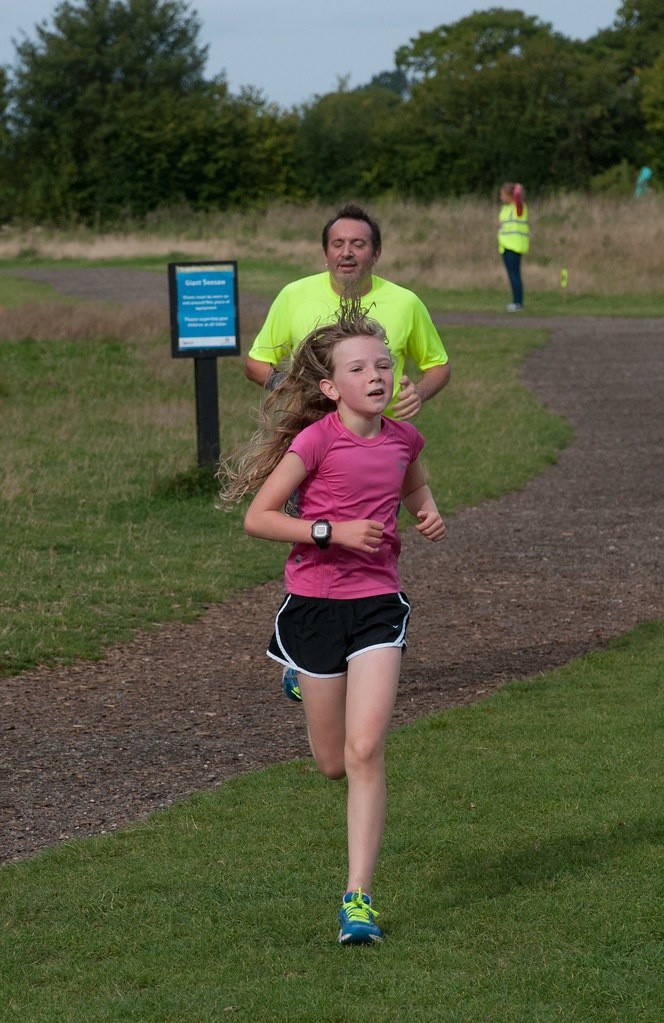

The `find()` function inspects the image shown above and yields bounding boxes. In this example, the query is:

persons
[495,182,532,311]
[237,317,449,953]
[243,200,455,708]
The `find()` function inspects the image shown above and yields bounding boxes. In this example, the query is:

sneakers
[281,665,302,702]
[339,890,384,944]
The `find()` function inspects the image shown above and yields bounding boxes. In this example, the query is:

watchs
[311,517,332,547]
